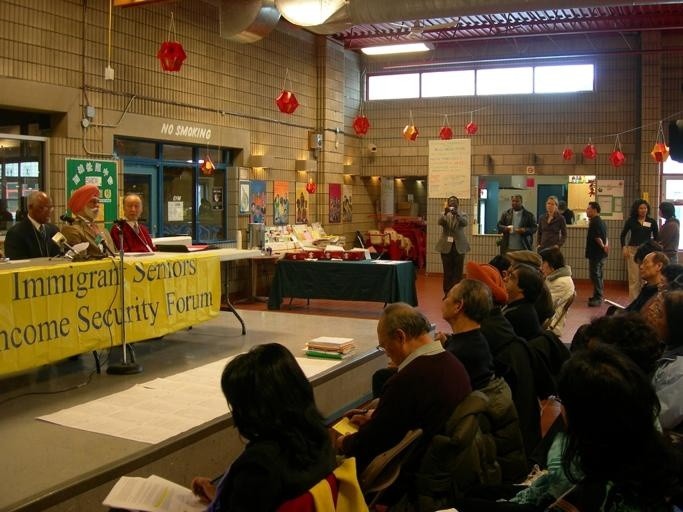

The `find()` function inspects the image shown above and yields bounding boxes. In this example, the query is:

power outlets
[86,106,95,117]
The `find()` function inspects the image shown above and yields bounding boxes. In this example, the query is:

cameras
[449,207,455,211]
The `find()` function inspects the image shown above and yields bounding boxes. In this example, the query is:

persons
[536,195,567,254]
[110,191,158,252]
[653,202,679,264]
[250,195,265,224]
[193,343,335,511]
[619,199,658,301]
[3,191,66,260]
[433,279,496,391]
[557,200,575,225]
[584,202,609,306]
[506,242,681,511]
[329,198,340,222]
[336,302,472,502]
[0,198,13,231]
[296,192,307,224]
[434,196,470,301]
[342,196,351,222]
[60,184,107,259]
[273,192,288,224]
[15,196,27,222]
[467,245,575,345]
[496,194,537,254]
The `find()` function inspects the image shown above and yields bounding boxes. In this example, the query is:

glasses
[376,336,391,353]
[38,205,54,213]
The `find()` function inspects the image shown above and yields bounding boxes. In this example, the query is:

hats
[505,250,542,266]
[466,261,508,303]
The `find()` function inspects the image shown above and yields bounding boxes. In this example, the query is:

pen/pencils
[361,408,368,414]
[195,473,224,496]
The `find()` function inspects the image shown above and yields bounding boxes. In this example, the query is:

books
[304,337,354,359]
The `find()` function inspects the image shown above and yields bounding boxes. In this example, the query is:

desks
[0,249,265,374]
[268,259,418,311]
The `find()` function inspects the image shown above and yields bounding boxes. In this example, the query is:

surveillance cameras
[368,144,377,153]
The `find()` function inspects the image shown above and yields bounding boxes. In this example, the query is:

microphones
[52,231,79,255]
[95,232,116,257]
[60,214,75,223]
[114,219,148,224]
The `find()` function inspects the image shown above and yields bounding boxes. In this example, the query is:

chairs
[360,293,683,512]
